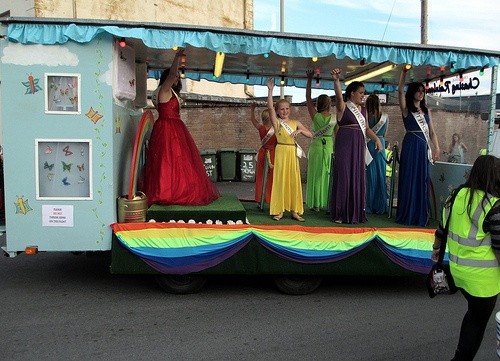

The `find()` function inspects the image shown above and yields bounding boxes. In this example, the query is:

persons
[251,102,278,210]
[433,155,500,361]
[365,93,390,214]
[305,69,337,211]
[396,66,441,225]
[267,77,314,222]
[385,140,394,201]
[442,133,468,164]
[328,67,383,225]
[138,49,222,206]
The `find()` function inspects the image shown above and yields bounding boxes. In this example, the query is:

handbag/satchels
[427,262,458,299]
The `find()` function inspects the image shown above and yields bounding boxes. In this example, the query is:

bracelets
[433,246,441,250]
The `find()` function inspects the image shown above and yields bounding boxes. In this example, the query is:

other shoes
[273,214,282,221]
[292,214,304,222]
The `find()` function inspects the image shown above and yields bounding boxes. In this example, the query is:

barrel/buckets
[117,195,147,224]
[495,311,500,359]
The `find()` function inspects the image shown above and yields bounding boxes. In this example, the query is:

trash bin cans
[219,147,239,182]
[201,148,219,183]
[239,147,258,182]
